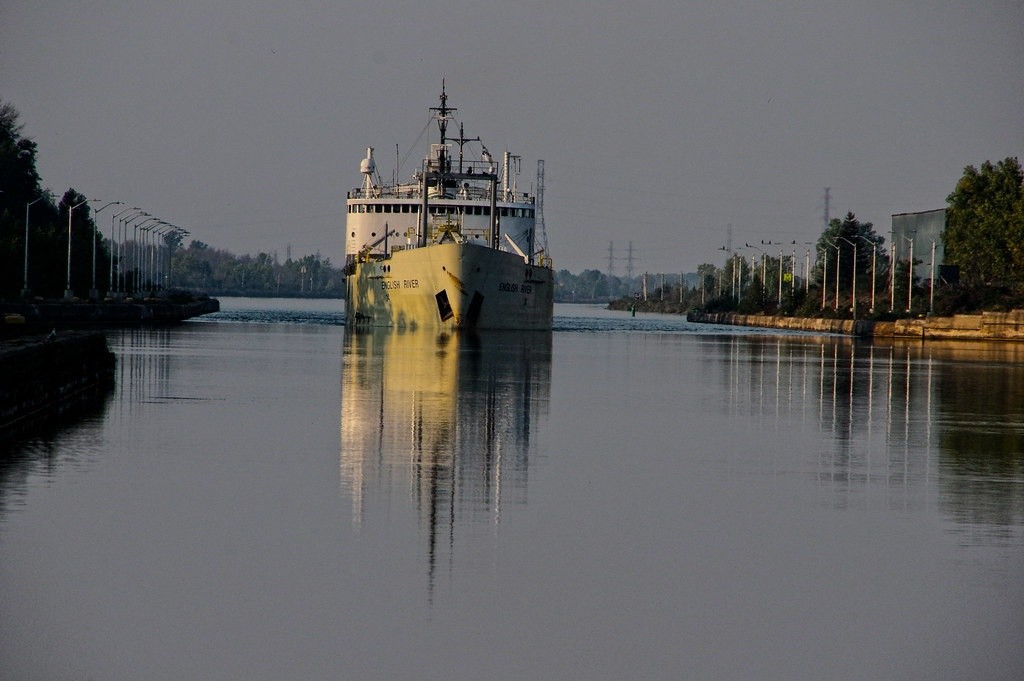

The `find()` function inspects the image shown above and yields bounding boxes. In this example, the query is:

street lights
[760,238,787,311]
[927,235,936,314]
[823,238,843,311]
[65,198,102,297]
[792,240,811,293]
[717,243,768,303]
[852,236,881,313]
[887,230,913,313]
[89,200,124,298]
[833,236,860,321]
[24,192,61,298]
[108,207,193,301]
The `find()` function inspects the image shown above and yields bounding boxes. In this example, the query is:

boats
[342,75,555,335]
